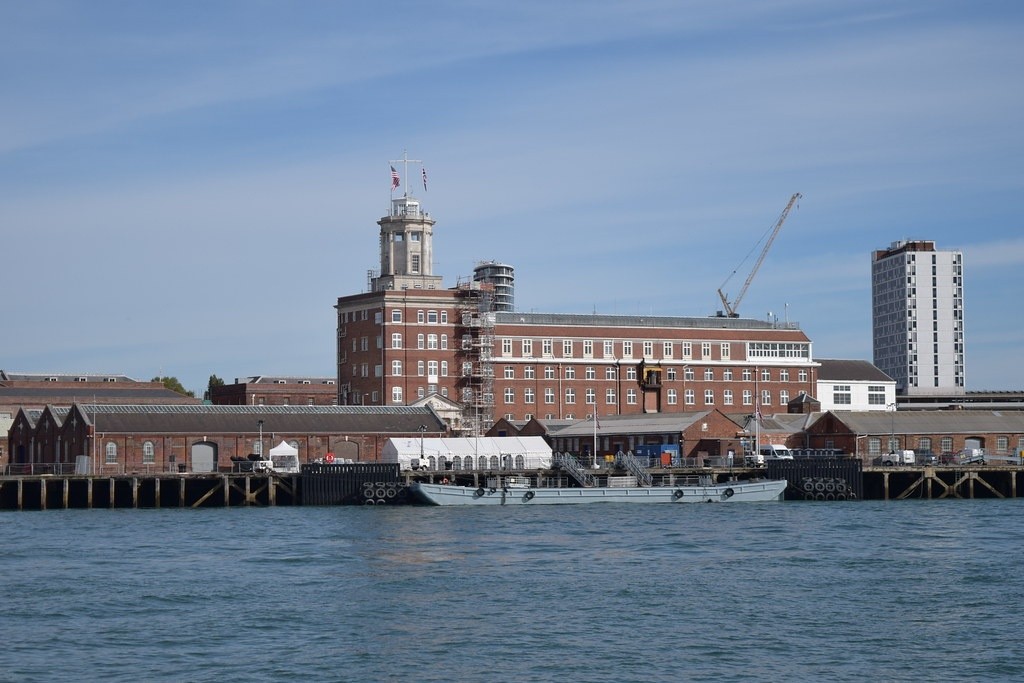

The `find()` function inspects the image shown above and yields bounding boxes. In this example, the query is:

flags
[390,166,400,191]
[423,168,427,190]
[757,401,763,421]
[596,409,600,428]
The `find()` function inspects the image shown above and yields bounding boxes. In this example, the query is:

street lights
[415,422,429,464]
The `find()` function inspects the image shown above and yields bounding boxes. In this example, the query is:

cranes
[711,187,808,321]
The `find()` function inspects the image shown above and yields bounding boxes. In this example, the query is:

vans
[760,443,793,464]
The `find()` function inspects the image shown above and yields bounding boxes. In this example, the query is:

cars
[939,451,958,464]
[909,448,939,465]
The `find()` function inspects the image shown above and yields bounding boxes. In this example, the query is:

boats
[411,475,788,506]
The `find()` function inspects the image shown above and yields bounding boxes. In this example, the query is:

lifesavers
[524,490,535,500]
[476,488,485,497]
[360,481,411,507]
[323,452,334,463]
[674,489,684,498]
[723,487,734,498]
[800,477,859,502]
